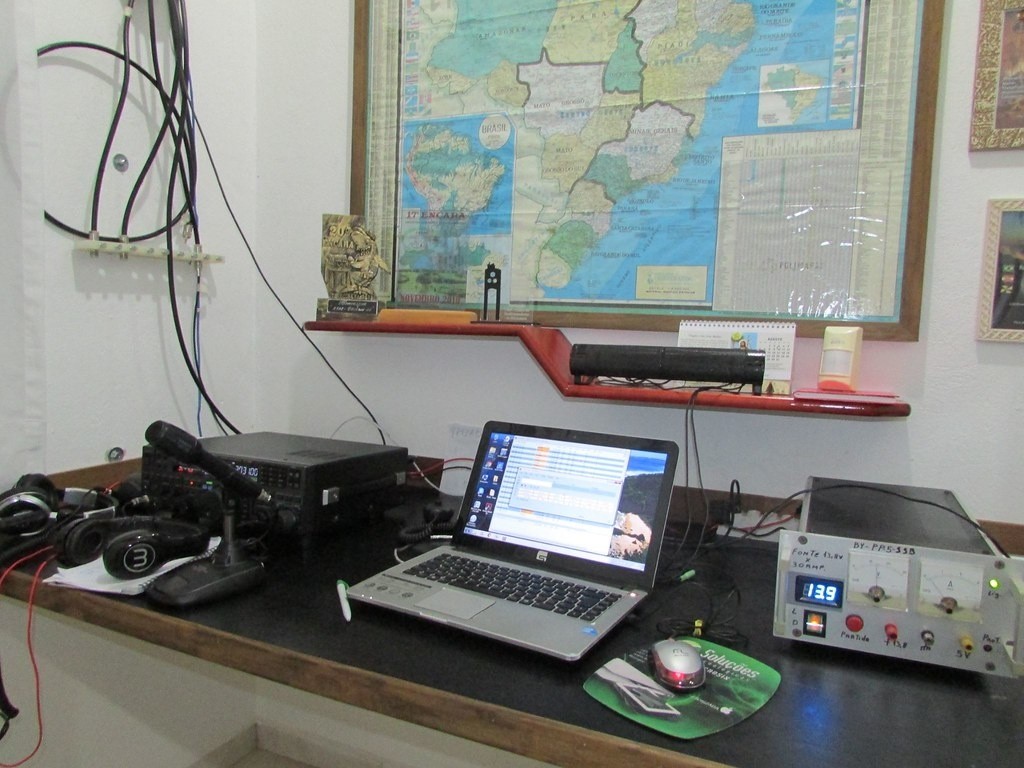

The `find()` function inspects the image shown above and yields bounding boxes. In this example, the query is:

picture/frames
[975,198,1024,344]
[968,0,1024,151]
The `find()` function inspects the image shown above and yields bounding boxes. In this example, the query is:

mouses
[649,637,705,689]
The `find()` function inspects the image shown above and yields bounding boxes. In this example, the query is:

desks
[0,458,1024,768]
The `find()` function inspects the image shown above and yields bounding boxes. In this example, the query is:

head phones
[54,514,205,578]
[0,472,114,541]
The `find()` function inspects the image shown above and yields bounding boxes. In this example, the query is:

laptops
[344,421,680,661]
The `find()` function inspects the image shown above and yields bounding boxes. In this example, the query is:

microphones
[144,420,272,503]
